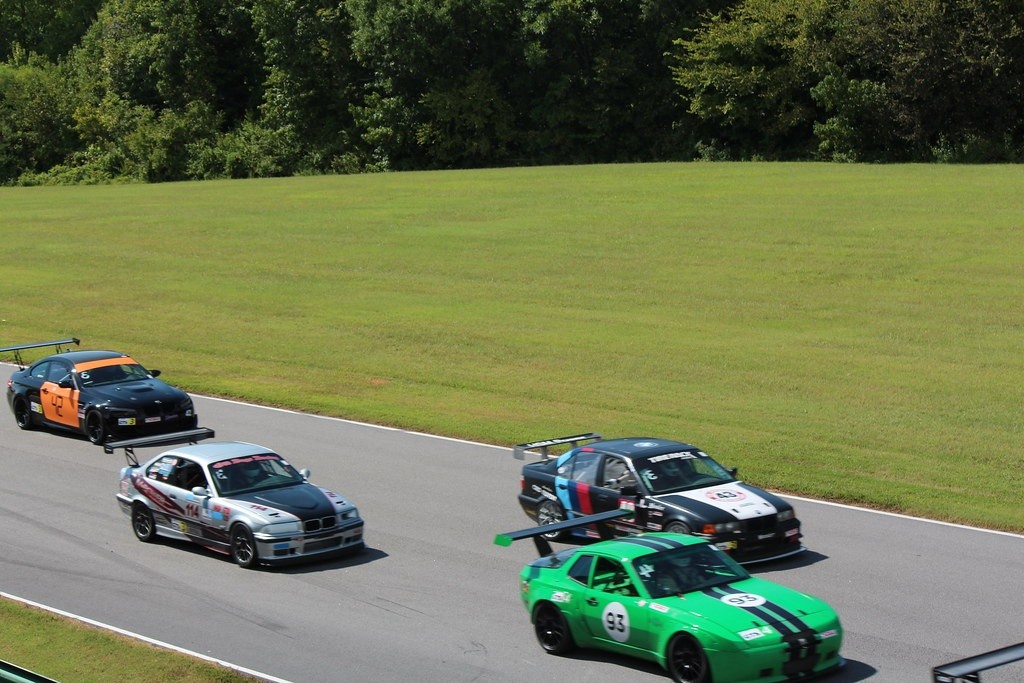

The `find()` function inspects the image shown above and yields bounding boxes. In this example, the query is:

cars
[96,427,365,568]
[495,511,848,683]
[513,431,810,567]
[1,336,199,447]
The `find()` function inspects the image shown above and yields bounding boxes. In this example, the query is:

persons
[656,458,690,488]
[236,460,268,490]
[657,554,706,594]
